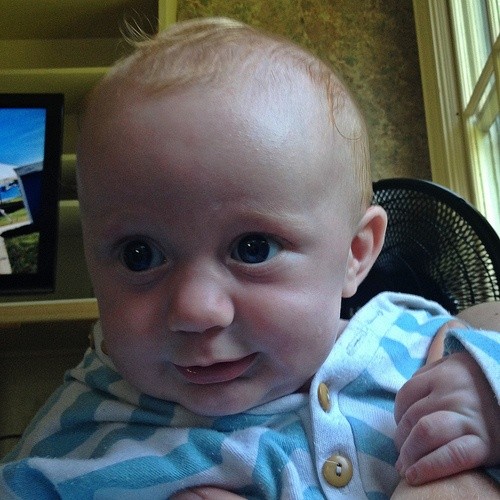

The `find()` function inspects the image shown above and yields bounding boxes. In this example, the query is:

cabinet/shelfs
[0,1,179,467]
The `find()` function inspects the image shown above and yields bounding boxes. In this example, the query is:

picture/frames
[0,90,67,299]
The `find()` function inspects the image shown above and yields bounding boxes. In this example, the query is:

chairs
[337,173,500,323]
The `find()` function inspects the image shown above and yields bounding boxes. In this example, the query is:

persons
[0,16,500,500]
